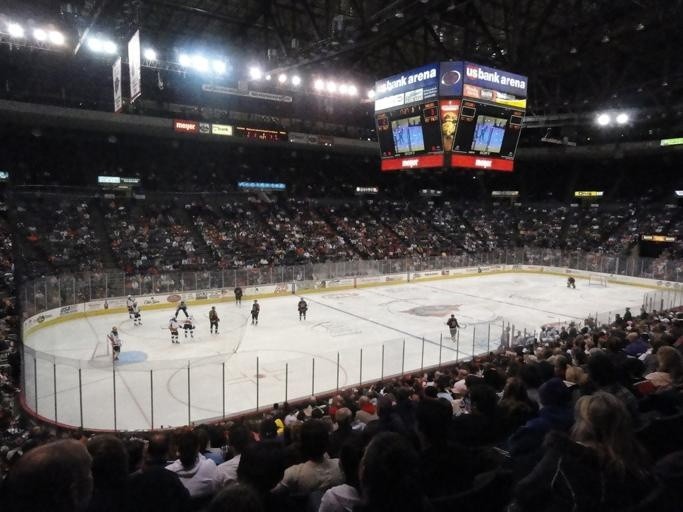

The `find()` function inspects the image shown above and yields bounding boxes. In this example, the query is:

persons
[0,123,683,512]
